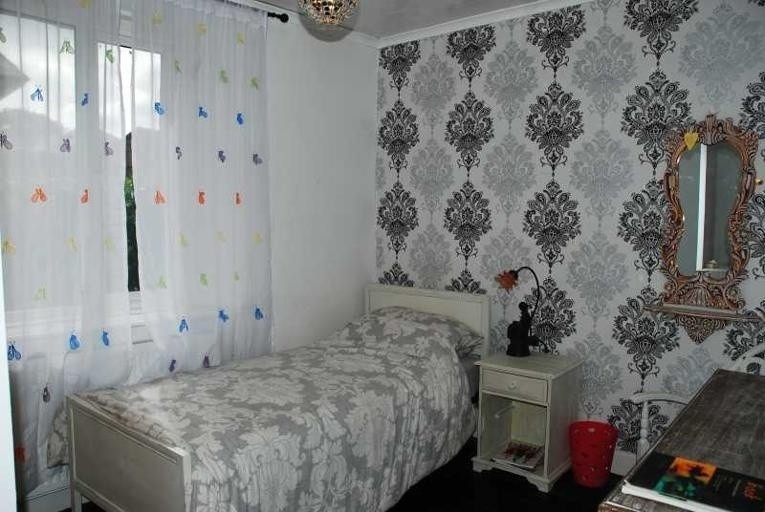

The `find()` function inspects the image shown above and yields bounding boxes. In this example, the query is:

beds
[65,282,491,512]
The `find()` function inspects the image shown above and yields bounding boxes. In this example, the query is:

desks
[599,369,765,512]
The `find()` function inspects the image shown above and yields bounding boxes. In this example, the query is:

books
[622,451,765,512]
[492,439,544,472]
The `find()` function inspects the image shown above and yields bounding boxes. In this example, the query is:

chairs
[632,344,765,462]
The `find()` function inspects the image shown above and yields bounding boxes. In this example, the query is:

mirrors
[643,112,760,321]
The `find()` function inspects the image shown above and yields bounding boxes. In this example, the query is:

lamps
[494,266,550,357]
[297,0,360,43]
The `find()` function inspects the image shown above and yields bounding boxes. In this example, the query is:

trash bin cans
[568,421,620,489]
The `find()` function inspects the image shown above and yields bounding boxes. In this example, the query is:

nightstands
[473,349,587,493]
